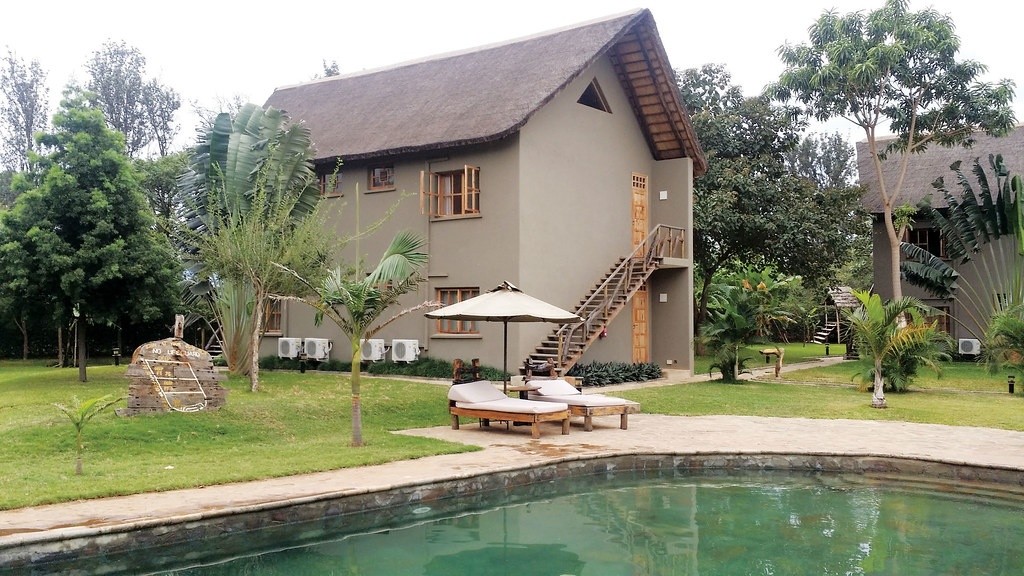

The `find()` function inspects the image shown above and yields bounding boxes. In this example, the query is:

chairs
[525,377,641,432]
[447,379,571,438]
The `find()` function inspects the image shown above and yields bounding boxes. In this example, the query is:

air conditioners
[278,337,302,358]
[359,339,385,361]
[959,338,981,354]
[391,339,419,362]
[304,338,329,359]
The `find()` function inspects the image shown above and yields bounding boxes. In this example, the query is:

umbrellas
[422,507,586,576]
[424,279,585,394]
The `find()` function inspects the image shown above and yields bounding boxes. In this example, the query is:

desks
[506,386,542,426]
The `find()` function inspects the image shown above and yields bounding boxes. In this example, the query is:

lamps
[825,343,829,348]
[575,377,583,385]
[765,353,770,358]
[113,348,119,354]
[1007,376,1016,383]
